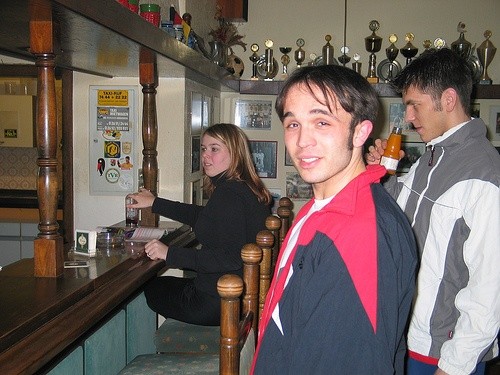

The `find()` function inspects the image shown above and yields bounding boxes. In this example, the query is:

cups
[125,198,139,226]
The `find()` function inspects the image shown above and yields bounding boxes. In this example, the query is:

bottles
[173,25,183,42]
[379,126,402,174]
[140,4,160,28]
[161,20,176,38]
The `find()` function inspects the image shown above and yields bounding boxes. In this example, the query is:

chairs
[118,197,291,375]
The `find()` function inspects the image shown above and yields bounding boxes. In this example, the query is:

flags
[169,6,190,46]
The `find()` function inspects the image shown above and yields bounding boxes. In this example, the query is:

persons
[127,124,274,326]
[243,103,476,198]
[246,65,419,375]
[117,156,133,170]
[365,50,500,375]
[182,13,209,58]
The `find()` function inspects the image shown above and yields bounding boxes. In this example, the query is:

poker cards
[63,228,98,269]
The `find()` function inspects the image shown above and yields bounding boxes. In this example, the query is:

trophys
[249,19,497,85]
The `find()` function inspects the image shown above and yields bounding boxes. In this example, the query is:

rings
[148,252,151,256]
